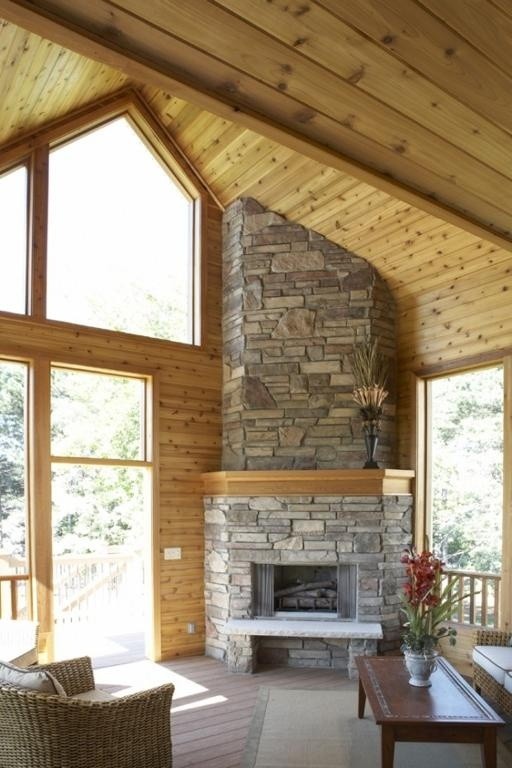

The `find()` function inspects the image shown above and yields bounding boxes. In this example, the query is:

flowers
[388,531,476,653]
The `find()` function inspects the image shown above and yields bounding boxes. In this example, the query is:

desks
[353,652,507,768]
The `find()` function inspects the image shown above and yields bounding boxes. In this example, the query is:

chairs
[1,655,173,767]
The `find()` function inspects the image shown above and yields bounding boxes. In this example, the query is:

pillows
[1,662,68,702]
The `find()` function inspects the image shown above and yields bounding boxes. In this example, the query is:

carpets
[238,681,512,768]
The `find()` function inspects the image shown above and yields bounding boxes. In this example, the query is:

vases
[402,645,440,688]
[361,419,381,469]
